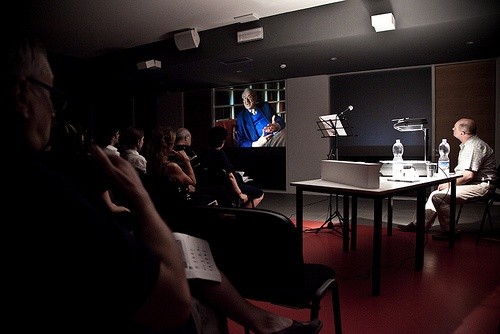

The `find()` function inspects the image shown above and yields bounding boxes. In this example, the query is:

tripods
[305,114,361,240]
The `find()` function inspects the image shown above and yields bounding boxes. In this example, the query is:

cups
[426,163,437,177]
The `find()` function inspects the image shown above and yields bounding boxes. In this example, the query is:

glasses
[24,75,69,110]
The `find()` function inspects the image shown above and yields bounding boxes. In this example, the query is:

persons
[0,42,323,334]
[396,118,497,241]
[236,88,286,147]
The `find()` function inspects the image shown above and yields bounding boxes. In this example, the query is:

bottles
[438,139,450,176]
[392,139,403,178]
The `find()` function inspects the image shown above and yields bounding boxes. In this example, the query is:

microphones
[341,106,354,114]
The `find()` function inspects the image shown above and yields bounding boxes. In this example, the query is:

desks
[289,173,464,297]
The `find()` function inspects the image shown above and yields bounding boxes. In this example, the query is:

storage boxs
[321,160,379,188]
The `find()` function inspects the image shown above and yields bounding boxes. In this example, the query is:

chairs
[132,140,341,334]
[456,164,500,246]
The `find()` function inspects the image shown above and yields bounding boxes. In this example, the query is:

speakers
[174,29,200,51]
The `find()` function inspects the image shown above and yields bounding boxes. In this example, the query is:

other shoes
[397,222,417,233]
[431,230,452,240]
[269,318,322,334]
